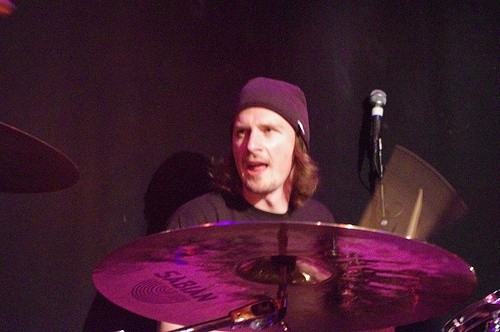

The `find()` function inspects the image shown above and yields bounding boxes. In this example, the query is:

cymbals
[0,122,80,196]
[91,221,477,332]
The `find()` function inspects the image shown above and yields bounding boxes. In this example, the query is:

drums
[441,288,500,332]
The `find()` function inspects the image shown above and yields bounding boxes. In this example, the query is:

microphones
[369,89,387,143]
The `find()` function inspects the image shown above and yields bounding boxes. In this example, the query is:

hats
[232,77,310,155]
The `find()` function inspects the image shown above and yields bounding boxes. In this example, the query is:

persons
[158,76,338,332]
